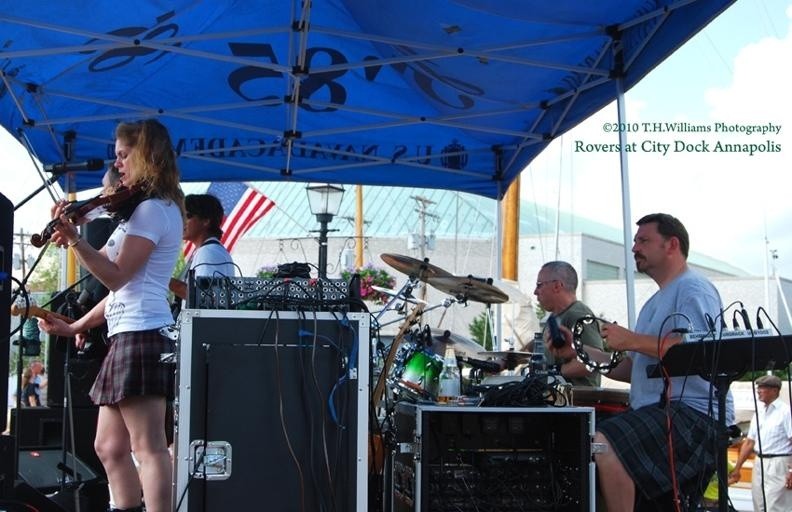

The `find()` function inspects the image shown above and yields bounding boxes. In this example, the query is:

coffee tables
[411,328,489,362]
[426,276,508,304]
[478,351,533,360]
[380,253,453,283]
[371,285,426,305]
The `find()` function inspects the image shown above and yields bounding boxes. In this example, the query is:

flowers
[42,158,106,176]
[65,288,90,327]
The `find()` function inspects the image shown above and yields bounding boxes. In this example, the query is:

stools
[758,454,789,458]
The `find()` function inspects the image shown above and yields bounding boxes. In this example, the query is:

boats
[10,406,95,452]
[17,450,99,497]
[79,219,118,295]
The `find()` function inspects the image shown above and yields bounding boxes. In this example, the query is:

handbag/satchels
[789,469,792,473]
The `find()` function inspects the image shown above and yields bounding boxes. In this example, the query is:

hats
[371,323,492,375]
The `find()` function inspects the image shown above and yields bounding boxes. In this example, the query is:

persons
[75,165,173,473]
[729,374,792,512]
[8,357,48,408]
[37,117,185,512]
[492,260,603,404]
[543,214,735,512]
[166,194,235,352]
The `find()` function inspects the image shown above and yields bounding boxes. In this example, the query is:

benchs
[183,179,274,264]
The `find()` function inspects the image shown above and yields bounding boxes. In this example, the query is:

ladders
[30,179,148,247]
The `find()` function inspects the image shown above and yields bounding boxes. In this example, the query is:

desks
[306,181,347,277]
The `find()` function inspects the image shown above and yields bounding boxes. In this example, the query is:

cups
[537,280,563,288]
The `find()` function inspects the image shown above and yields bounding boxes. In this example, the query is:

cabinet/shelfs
[531,332,549,395]
[437,344,461,401]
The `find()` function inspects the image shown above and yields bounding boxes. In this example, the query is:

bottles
[756,375,781,388]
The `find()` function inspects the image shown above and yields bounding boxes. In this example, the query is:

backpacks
[397,343,446,395]
